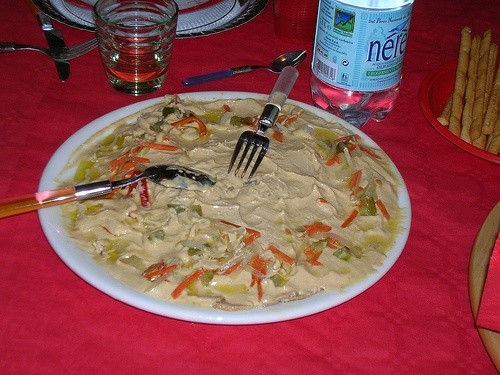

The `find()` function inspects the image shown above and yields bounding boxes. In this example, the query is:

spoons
[0,166,217,218]
[183,50,306,86]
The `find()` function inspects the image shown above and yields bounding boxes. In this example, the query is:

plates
[30,0,270,39]
[38,91,411,325]
[418,60,500,164]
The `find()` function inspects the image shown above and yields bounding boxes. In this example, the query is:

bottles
[311,0,414,126]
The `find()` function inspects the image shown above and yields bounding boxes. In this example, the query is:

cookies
[436,25,500,156]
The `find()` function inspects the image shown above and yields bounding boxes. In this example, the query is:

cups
[94,0,179,96]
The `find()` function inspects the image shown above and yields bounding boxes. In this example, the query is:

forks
[0,38,98,60]
[228,66,299,183]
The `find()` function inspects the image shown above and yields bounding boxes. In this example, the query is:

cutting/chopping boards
[469,203,500,373]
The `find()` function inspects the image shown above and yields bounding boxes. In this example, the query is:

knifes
[38,12,71,82]
